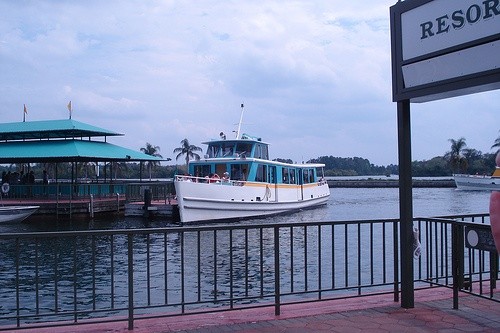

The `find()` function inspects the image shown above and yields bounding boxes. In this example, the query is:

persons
[178,174,192,182]
[207,174,220,183]
[220,132,224,139]
[223,172,230,183]
[2,171,35,199]
[43,170,49,200]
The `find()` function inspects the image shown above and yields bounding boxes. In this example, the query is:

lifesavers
[2,183,9,193]
[243,169,247,173]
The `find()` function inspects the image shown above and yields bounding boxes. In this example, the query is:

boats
[453,174,500,190]
[172,138,331,225]
[0,205,41,224]
